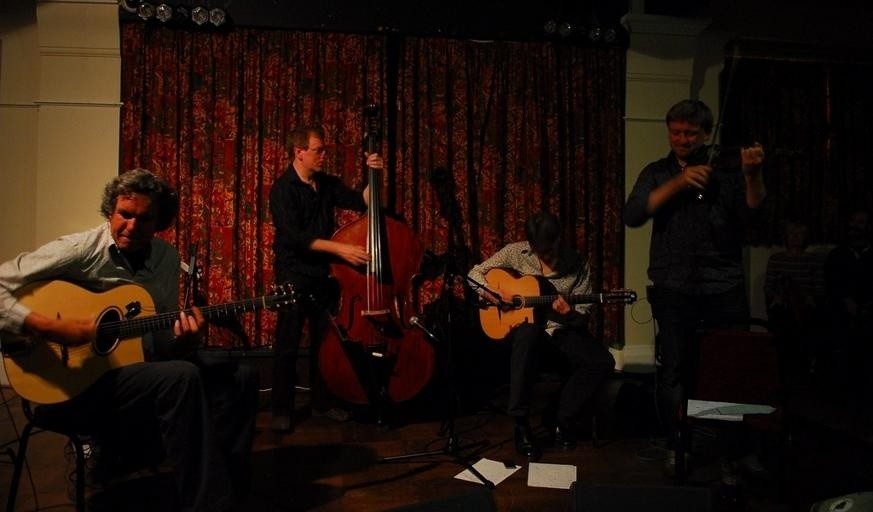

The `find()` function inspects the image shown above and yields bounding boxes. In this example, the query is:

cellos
[319,105,436,402]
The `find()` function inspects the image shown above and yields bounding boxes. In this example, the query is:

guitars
[2,280,297,404]
[479,267,637,339]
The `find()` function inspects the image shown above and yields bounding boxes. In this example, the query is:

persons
[825,212,873,315]
[765,223,825,328]
[622,99,767,395]
[269,128,383,434]
[0,168,259,512]
[466,214,616,458]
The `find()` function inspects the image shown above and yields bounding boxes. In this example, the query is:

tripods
[373,422,495,489]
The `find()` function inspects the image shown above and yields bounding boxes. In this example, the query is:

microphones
[409,316,439,342]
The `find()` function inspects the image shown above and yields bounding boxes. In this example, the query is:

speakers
[719,61,827,149]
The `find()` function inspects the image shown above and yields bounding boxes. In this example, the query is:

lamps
[539,16,622,49]
[118,0,232,26]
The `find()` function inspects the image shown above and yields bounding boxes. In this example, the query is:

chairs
[6,396,103,511]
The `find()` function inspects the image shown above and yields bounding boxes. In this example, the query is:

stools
[505,353,575,433]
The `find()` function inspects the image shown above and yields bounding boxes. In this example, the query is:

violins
[693,143,784,180]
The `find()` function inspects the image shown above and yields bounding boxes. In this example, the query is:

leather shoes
[549,427,570,451]
[515,429,539,460]
